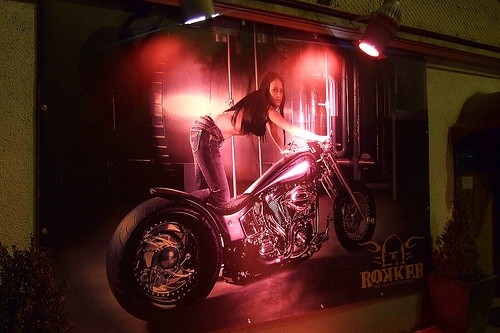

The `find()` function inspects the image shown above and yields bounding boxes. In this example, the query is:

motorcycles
[105,138,378,320]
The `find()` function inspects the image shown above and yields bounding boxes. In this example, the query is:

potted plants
[424,194,499,330]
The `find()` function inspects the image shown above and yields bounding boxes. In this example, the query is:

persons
[184,70,333,210]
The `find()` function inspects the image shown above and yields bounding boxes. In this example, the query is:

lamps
[180,0,217,26]
[351,0,403,59]
